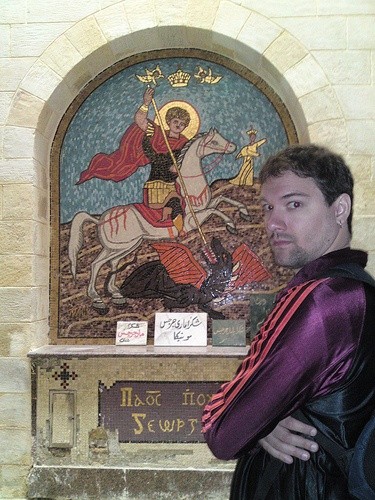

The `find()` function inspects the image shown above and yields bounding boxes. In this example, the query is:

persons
[201,144,375,500]
